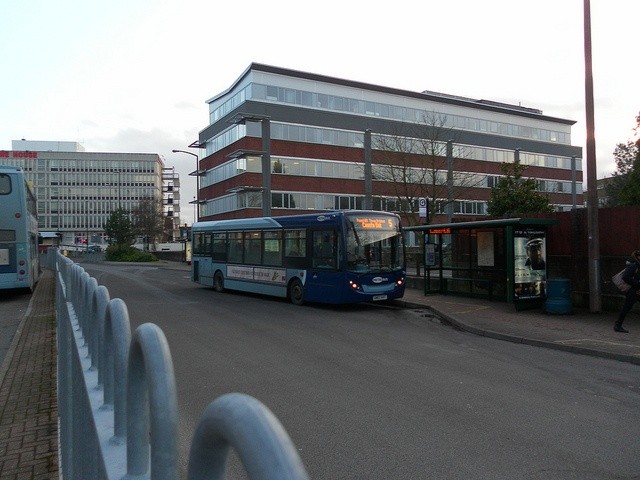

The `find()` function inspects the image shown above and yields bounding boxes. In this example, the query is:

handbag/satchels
[612,267,632,292]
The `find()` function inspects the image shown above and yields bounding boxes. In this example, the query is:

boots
[614,321,628,333]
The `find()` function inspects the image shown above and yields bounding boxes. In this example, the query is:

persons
[527,285,535,296]
[614,250,640,333]
[525,237,545,270]
[515,283,524,296]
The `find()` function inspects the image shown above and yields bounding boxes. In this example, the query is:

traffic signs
[419,198,427,217]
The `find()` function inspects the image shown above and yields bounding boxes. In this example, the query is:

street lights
[173,150,199,222]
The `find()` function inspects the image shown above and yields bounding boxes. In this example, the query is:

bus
[191,210,407,306]
[0,166,39,293]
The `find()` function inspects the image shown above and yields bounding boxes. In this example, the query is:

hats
[524,238,544,248]
